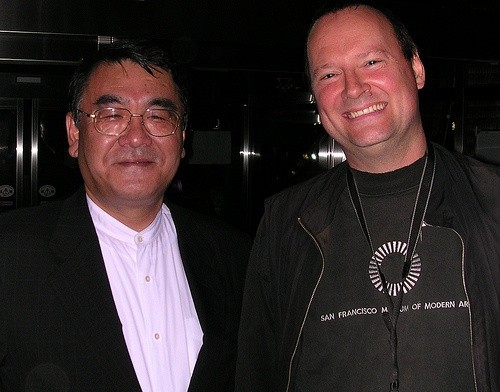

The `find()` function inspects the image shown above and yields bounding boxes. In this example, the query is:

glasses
[75,106,187,138]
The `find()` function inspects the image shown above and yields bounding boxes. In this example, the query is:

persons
[1,38,257,391]
[233,1,500,391]
